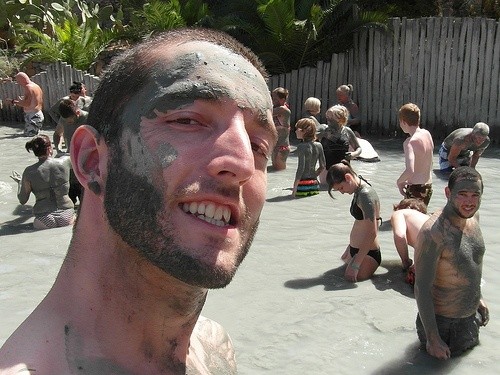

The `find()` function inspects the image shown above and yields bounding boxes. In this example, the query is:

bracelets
[351,262,360,271]
[408,259,414,268]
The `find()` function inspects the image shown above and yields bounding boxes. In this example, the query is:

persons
[9,134,75,229]
[270,87,291,169]
[438,121,491,174]
[414,166,489,359]
[292,97,362,198]
[396,102,433,206]
[0,29,279,375]
[391,197,429,278]
[336,84,361,127]
[54,81,93,202]
[5,71,44,137]
[326,159,382,282]
